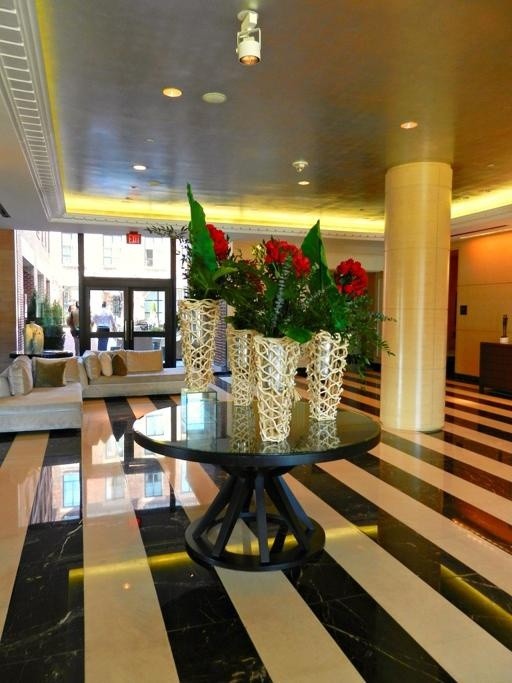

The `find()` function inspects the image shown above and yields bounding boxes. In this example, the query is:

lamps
[235,11,262,65]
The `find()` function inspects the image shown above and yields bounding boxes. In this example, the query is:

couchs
[78,350,203,398]
[0,357,82,433]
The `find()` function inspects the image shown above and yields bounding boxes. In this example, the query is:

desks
[133,401,381,571]
[480,342,512,396]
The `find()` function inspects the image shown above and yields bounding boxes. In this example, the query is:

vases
[174,298,351,443]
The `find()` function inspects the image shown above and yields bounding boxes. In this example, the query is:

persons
[66,301,117,351]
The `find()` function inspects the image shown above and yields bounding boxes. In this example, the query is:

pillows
[9,352,129,397]
